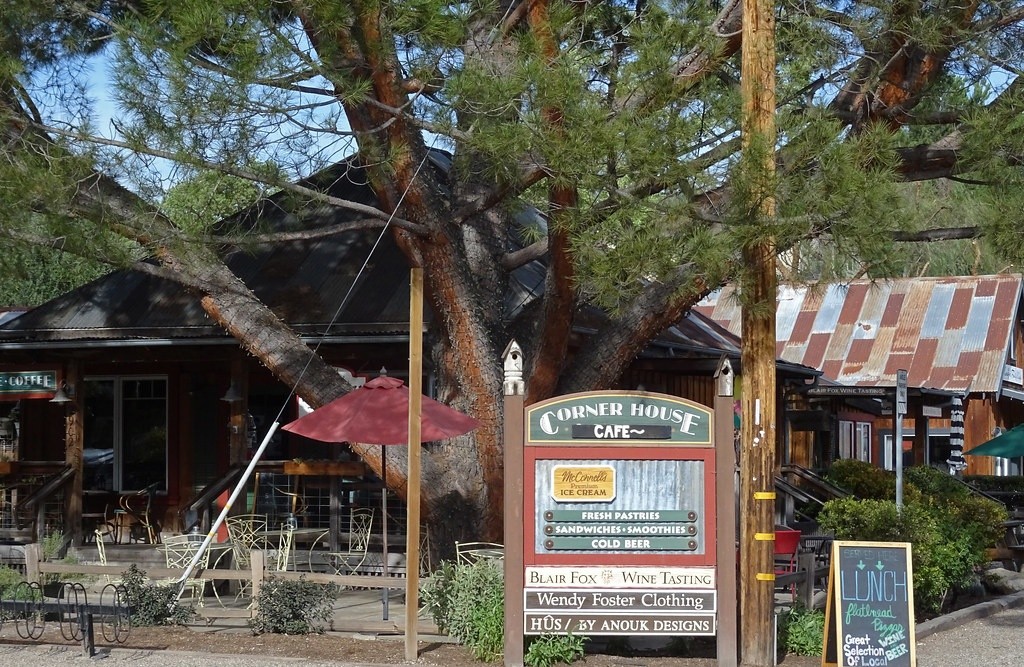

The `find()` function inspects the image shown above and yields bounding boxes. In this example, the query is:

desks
[82,489,119,545]
[156,543,236,607]
[257,527,330,572]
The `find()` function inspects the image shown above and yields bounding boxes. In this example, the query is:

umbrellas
[946,393,968,476]
[281,366,480,620]
[960,424,1024,459]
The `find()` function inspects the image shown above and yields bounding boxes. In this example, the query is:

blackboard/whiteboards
[833,540,916,667]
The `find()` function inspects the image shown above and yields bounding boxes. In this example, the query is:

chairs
[111,481,162,544]
[225,513,293,610]
[57,491,114,544]
[316,506,371,594]
[93,530,149,597]
[454,540,505,575]
[774,531,802,603]
[156,534,212,616]
